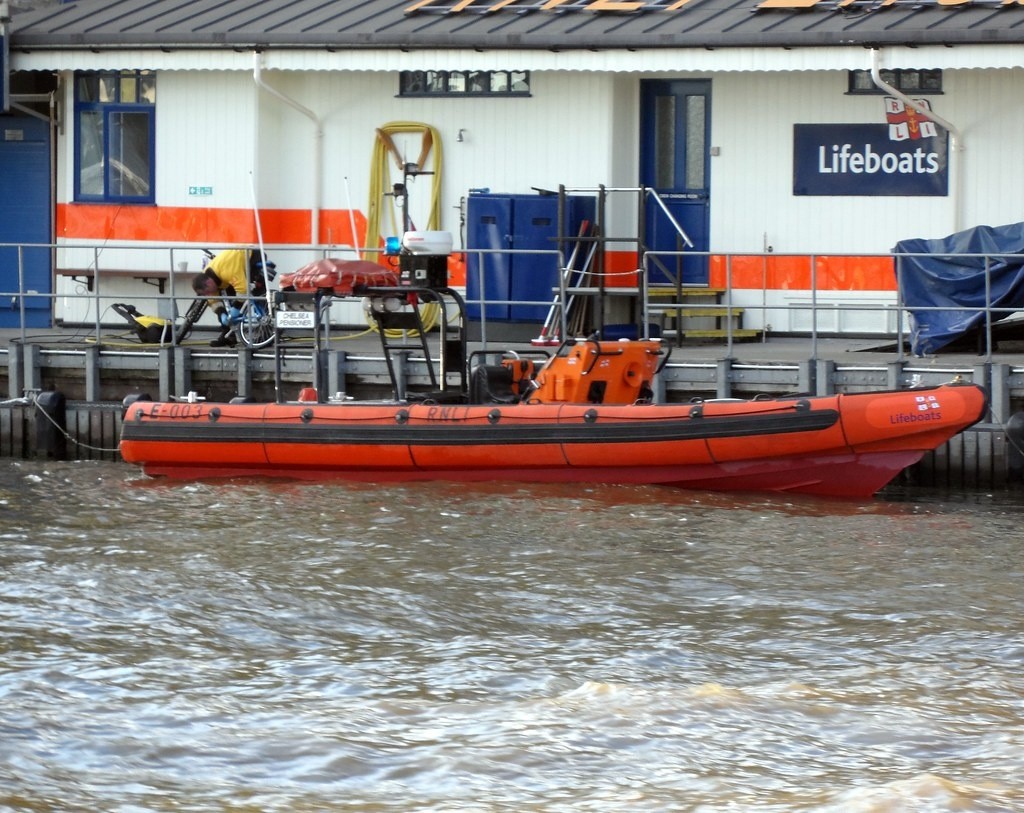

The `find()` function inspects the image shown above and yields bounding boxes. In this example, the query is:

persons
[192,243,267,346]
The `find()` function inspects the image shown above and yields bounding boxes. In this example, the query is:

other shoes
[210,332,237,346]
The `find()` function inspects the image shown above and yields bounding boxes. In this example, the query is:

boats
[117,142,986,502]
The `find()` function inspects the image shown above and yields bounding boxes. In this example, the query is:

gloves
[220,313,229,324]
[229,307,240,319]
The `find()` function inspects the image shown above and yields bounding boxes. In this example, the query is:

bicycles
[177,248,286,348]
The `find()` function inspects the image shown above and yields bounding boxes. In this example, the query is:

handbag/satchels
[112,302,172,343]
